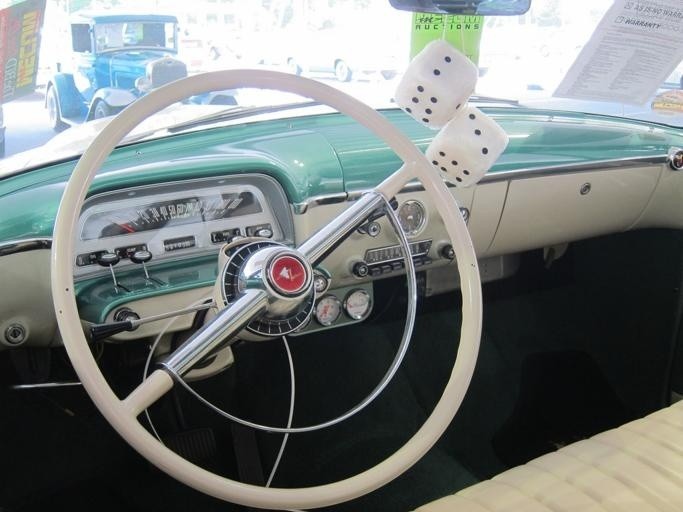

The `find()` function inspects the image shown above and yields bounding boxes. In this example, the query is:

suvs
[44,6,237,133]
